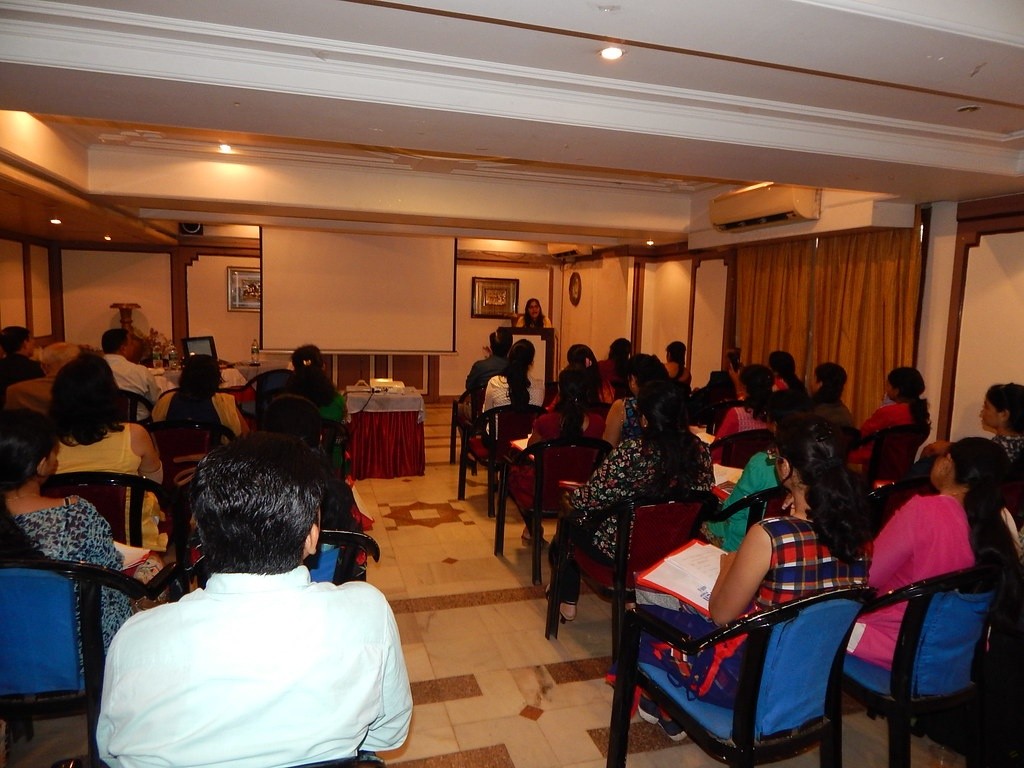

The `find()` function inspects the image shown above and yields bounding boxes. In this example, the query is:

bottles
[169,344,176,352]
[251,339,259,363]
[153,343,163,368]
[169,348,178,375]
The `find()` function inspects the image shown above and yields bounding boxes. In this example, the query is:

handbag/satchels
[345,478,374,531]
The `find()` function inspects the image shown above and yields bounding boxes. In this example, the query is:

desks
[146,361,289,389]
[344,385,427,481]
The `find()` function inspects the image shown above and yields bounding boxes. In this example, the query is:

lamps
[646,237,654,246]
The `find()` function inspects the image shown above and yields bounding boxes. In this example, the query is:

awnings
[0,326,375,726]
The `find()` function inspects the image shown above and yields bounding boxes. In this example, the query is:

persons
[95,433,414,768]
[636,411,872,741]
[466,329,1024,669]
[515,298,552,328]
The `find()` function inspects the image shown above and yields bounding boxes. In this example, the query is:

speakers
[178,223,203,235]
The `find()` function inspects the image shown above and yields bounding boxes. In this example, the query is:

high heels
[545,583,577,624]
[521,535,549,546]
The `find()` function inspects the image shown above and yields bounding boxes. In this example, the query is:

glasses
[767,447,782,459]
[218,376,225,384]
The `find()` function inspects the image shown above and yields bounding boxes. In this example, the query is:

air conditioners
[708,182,822,233]
[547,242,592,259]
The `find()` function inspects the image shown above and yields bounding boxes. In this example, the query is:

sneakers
[638,697,687,741]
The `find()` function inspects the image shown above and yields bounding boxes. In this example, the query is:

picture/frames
[181,335,218,359]
[227,266,260,312]
[471,275,520,320]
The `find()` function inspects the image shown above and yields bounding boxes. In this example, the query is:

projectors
[369,378,406,394]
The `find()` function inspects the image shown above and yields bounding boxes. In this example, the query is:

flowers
[135,328,176,361]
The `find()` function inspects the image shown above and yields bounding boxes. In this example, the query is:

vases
[109,302,145,364]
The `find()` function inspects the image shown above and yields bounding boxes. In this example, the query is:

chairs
[190,526,381,591]
[40,472,193,592]
[118,388,154,429]
[0,558,387,768]
[143,419,236,454]
[450,386,1024,768]
[234,368,294,422]
